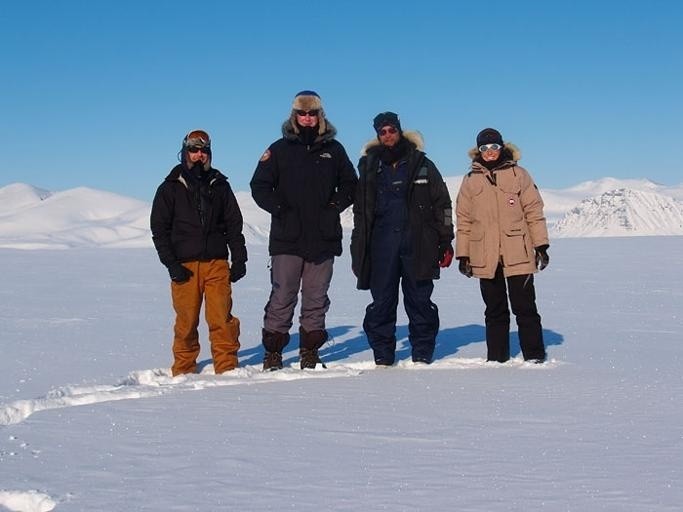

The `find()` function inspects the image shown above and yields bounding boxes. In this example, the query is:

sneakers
[299,349,329,370]
[261,350,283,371]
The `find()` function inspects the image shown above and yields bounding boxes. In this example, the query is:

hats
[180,129,213,171]
[477,128,503,146]
[373,111,399,129]
[293,90,327,134]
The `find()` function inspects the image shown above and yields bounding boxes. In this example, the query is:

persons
[147,129,248,379]
[348,111,456,368]
[249,90,358,372]
[453,125,550,367]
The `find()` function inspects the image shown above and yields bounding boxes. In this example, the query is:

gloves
[167,263,194,284]
[457,259,474,278]
[228,261,247,284]
[535,247,549,271]
[438,247,454,268]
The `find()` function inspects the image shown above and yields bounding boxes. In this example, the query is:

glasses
[294,108,318,117]
[479,142,503,153]
[378,127,398,136]
[188,146,209,154]
